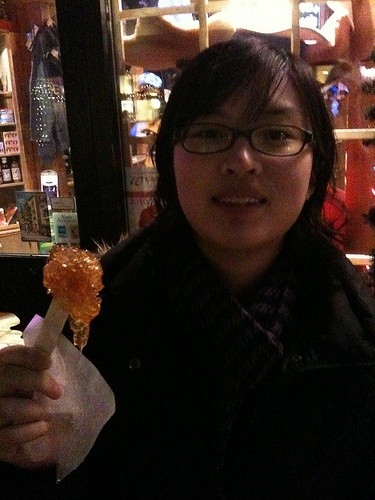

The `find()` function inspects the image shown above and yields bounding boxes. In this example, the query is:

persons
[0,37,375,500]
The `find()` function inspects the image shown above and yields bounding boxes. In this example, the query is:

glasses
[172,122,315,158]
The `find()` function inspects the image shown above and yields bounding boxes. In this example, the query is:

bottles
[0,157,21,184]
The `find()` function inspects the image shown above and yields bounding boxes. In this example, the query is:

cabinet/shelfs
[119,63,149,167]
[0,30,73,256]
